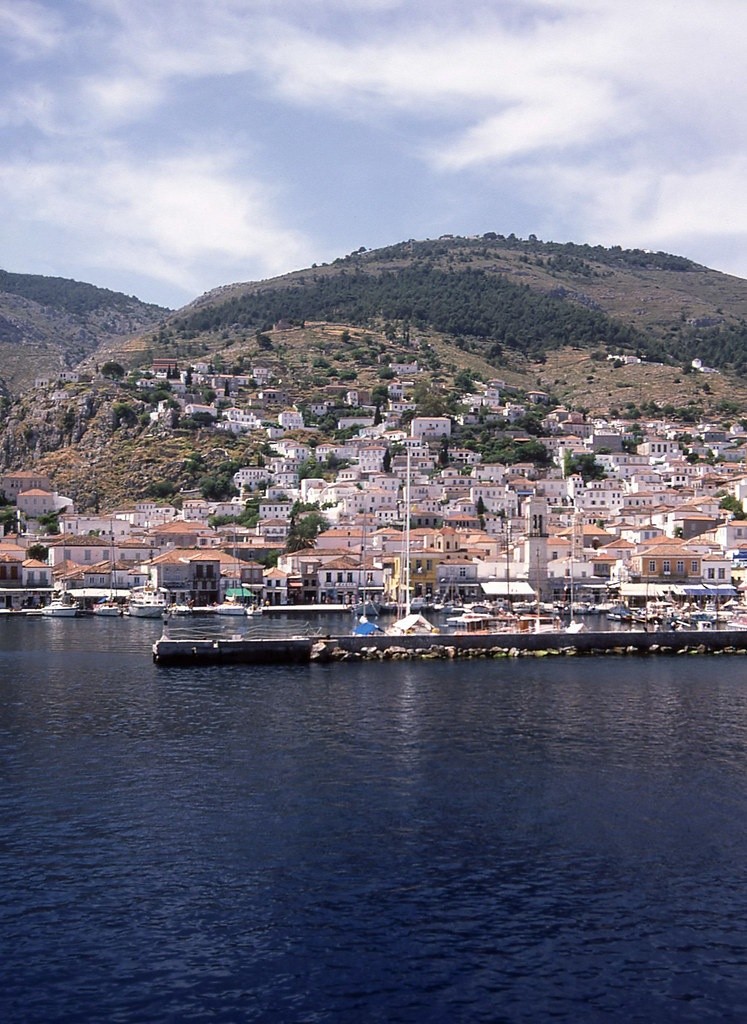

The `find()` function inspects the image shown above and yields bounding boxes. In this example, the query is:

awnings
[617,583,738,597]
[226,588,256,597]
[480,581,536,595]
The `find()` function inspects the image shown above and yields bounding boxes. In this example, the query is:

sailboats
[339,441,747,635]
[213,520,263,616]
[40,516,193,617]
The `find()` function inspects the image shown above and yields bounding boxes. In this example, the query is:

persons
[266,600,270,607]
[652,614,683,631]
